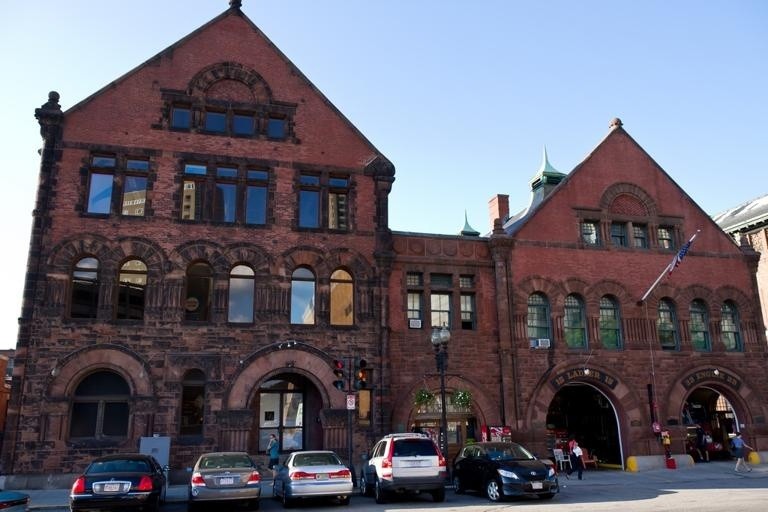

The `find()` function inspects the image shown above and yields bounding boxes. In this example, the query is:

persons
[730,433,754,473]
[687,431,713,461]
[266,434,280,486]
[565,433,585,480]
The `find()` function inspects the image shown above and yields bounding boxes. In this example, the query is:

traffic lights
[353,359,369,390]
[331,355,348,393]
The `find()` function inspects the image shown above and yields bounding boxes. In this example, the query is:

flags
[669,239,690,277]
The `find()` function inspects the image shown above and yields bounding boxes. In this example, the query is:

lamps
[51,358,60,376]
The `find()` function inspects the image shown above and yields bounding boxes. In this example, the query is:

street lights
[429,321,454,486]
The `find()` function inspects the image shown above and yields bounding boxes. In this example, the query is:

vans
[360,431,448,504]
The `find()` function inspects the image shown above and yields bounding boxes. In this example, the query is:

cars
[68,453,172,512]
[452,440,560,503]
[0,488,30,511]
[186,451,262,512]
[271,450,354,508]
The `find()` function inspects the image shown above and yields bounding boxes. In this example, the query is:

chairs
[552,448,572,472]
[580,448,599,471]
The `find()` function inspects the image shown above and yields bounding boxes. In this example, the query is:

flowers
[413,388,436,407]
[450,388,472,408]
